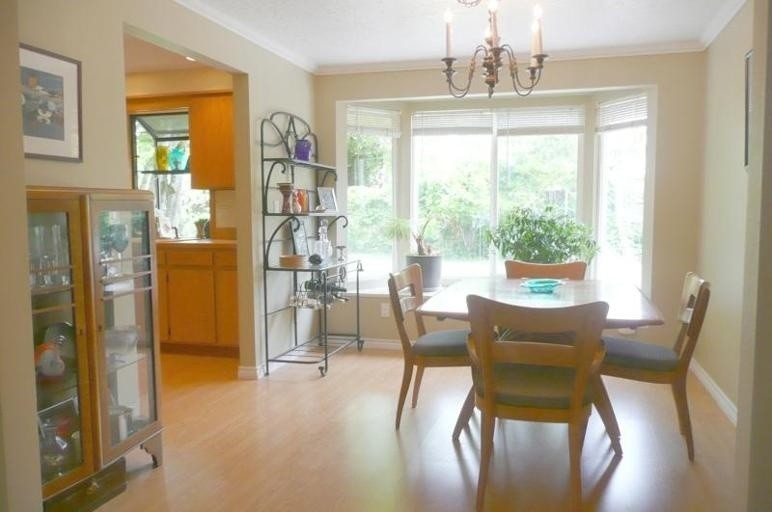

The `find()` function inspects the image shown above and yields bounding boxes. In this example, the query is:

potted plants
[388,207,468,292]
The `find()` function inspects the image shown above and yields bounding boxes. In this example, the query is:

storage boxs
[43,456,126,512]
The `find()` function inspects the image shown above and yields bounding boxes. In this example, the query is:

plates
[42,320,79,363]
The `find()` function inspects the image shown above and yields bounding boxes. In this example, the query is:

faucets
[170,226,180,239]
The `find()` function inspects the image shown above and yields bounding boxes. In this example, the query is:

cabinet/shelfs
[212,249,239,358]
[166,249,214,356]
[27,190,163,512]
[262,158,364,377]
[191,94,234,190]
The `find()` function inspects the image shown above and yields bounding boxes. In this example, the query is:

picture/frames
[316,187,338,212]
[289,221,310,256]
[20,43,83,164]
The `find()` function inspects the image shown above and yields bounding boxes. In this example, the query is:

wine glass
[98,222,133,277]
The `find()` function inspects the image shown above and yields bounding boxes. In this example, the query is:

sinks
[158,237,199,243]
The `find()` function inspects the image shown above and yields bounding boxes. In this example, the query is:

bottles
[289,280,351,311]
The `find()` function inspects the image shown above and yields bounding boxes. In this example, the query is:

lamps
[435,2,547,101]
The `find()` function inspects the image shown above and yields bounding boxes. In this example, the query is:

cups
[29,224,69,288]
